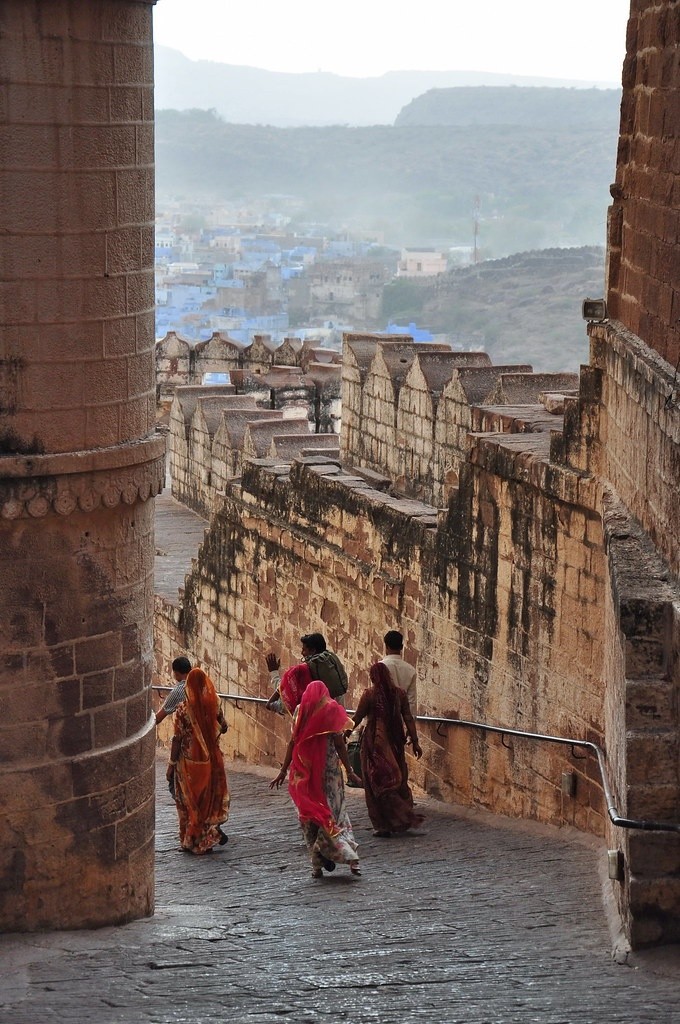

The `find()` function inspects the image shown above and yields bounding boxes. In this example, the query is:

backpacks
[301,650,348,699]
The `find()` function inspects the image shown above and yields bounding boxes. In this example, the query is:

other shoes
[350,861,361,876]
[372,830,391,837]
[217,828,228,845]
[312,869,323,877]
[178,846,191,852]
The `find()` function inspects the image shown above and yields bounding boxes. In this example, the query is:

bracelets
[280,769,287,776]
[346,766,354,776]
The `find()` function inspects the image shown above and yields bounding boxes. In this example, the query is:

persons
[269,680,364,878]
[379,630,418,746]
[166,668,228,854]
[265,633,348,707]
[266,663,312,714]
[342,662,422,838]
[155,657,193,724]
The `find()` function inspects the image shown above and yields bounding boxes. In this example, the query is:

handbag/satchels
[346,731,364,787]
